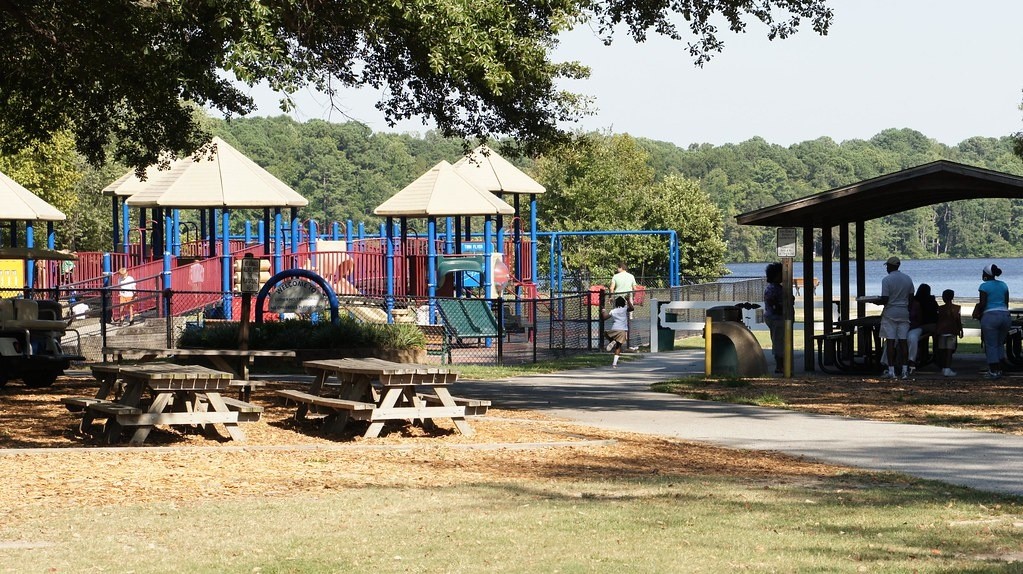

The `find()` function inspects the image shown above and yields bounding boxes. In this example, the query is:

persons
[881,284,936,376]
[609,263,637,341]
[72,295,89,320]
[975,263,1012,379]
[118,268,136,326]
[62,260,75,283]
[602,296,634,368]
[874,257,915,380]
[69,286,76,303]
[764,262,795,373]
[937,289,963,377]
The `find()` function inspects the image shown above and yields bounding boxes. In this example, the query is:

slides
[436,298,507,348]
[324,277,394,324]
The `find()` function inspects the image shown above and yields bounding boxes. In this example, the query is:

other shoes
[942,367,957,376]
[882,372,897,380]
[984,370,1002,378]
[606,340,616,351]
[775,366,783,373]
[909,366,916,375]
[612,364,617,369]
[902,369,908,380]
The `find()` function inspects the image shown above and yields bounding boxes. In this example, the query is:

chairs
[0,298,14,330]
[15,299,38,320]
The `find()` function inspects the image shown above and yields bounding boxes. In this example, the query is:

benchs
[809,332,850,371]
[60,396,146,426]
[1007,329,1019,364]
[274,388,377,423]
[376,390,491,417]
[196,393,264,423]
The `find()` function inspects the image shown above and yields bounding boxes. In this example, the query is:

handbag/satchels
[769,297,783,316]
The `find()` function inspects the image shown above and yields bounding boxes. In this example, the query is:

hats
[884,256,901,266]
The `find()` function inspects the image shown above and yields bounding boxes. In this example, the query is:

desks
[1007,310,1023,373]
[100,345,295,402]
[295,358,474,438]
[827,316,884,376]
[81,361,247,444]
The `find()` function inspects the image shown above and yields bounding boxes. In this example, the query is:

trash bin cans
[706,306,743,324]
[658,301,678,352]
